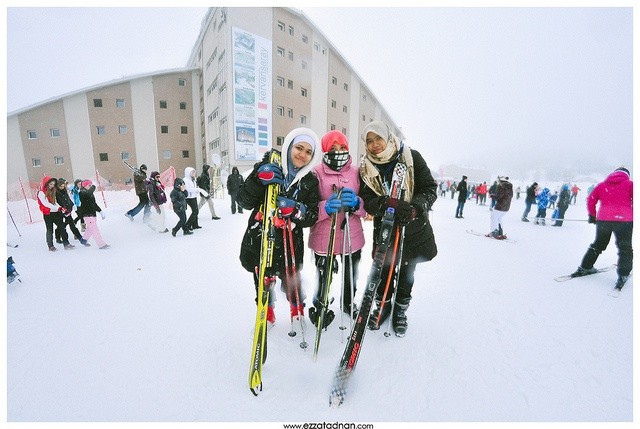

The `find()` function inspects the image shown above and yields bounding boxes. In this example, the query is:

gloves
[587,216,596,224]
[341,188,359,208]
[382,196,410,223]
[324,193,342,214]
[276,195,306,223]
[257,163,284,187]
[100,210,106,220]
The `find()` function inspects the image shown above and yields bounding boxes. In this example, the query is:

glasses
[156,175,160,179]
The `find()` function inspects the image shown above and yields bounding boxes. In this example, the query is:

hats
[81,180,92,187]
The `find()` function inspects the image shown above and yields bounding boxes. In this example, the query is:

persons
[439,180,456,199]
[521,182,538,222]
[308,131,367,318]
[170,177,193,237]
[71,180,88,232]
[480,182,488,204]
[234,127,320,332]
[357,122,438,336]
[124,164,151,223]
[569,184,581,205]
[476,183,482,203]
[517,187,520,198]
[553,185,569,226]
[79,180,110,249]
[37,176,75,251]
[148,171,168,232]
[455,176,467,219]
[55,180,91,247]
[468,184,475,200]
[580,167,634,281]
[548,192,558,210]
[227,167,245,214]
[196,164,222,220]
[488,175,513,238]
[536,188,549,225]
[182,166,202,231]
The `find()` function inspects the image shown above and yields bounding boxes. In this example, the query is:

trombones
[248,148,282,396]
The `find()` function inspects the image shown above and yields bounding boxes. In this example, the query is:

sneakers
[392,311,408,333]
[266,305,276,324]
[185,223,193,230]
[581,266,597,274]
[212,216,221,220]
[291,305,304,318]
[184,230,193,235]
[344,304,360,319]
[171,228,176,237]
[192,226,202,229]
[368,306,388,327]
